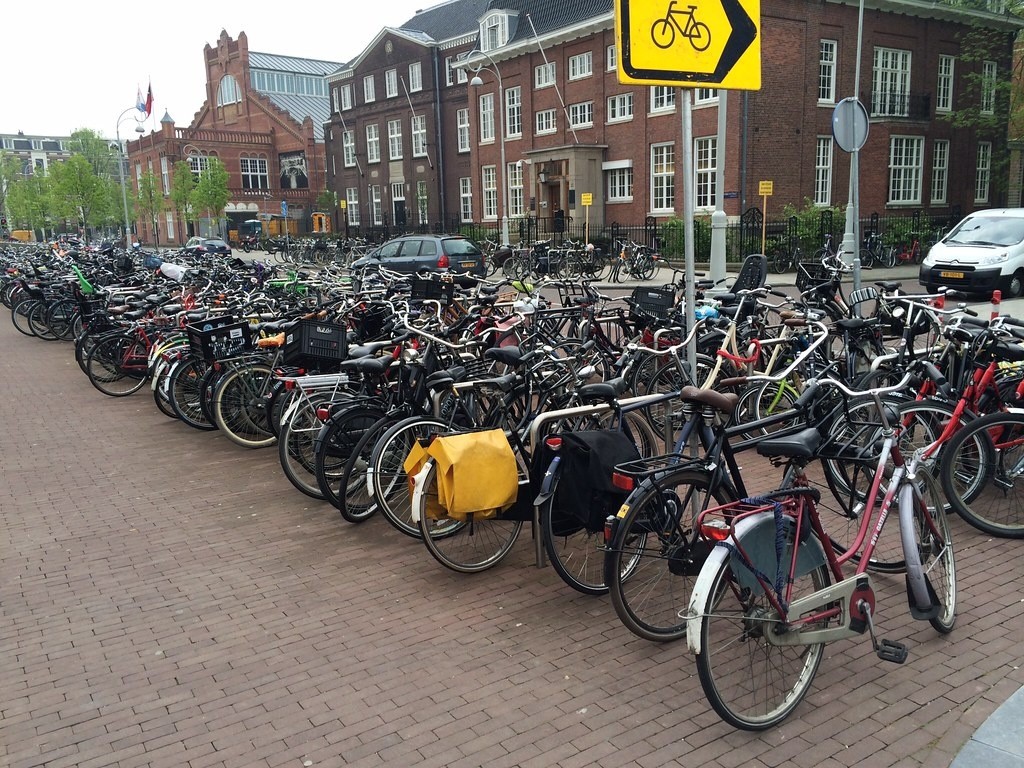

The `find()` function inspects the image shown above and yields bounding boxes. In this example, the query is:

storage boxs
[628,287,675,325]
[794,262,841,295]
[186,314,254,364]
[284,319,346,368]
[143,255,163,269]
[411,281,443,300]
[117,257,133,271]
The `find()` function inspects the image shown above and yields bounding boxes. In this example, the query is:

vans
[348,233,487,289]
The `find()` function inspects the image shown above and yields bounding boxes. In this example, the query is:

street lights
[465,49,511,247]
[183,144,215,239]
[25,160,44,244]
[116,107,149,253]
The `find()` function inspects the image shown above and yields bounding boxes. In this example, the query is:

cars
[918,206,1023,300]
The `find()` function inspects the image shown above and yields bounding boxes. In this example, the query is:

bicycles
[0,226,1023,732]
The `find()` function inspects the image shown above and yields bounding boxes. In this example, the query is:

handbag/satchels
[403,428,519,536]
[551,431,643,549]
